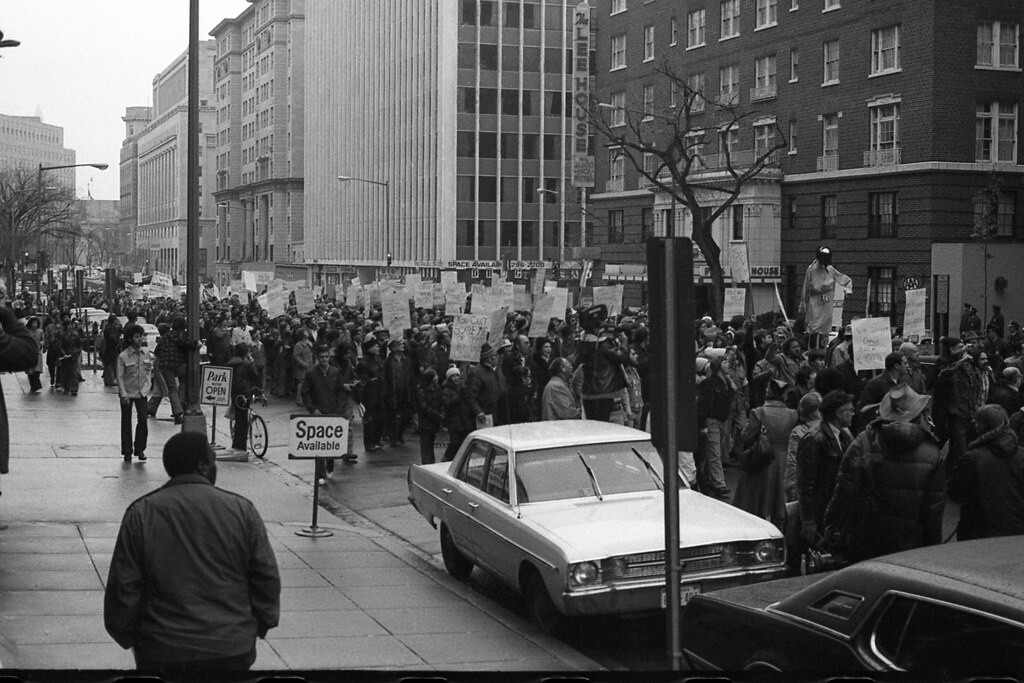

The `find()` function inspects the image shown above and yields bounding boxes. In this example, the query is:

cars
[407,420,787,630]
[71,305,161,356]
[680,535,1024,672]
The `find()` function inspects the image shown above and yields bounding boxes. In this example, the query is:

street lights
[10,187,56,295]
[338,176,389,252]
[537,187,560,267]
[36,164,108,311]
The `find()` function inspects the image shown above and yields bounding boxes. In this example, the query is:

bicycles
[230,393,268,458]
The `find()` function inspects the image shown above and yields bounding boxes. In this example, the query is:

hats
[963,330,986,344]
[987,323,1001,330]
[496,339,512,356]
[844,324,853,336]
[818,388,856,414]
[373,326,389,335]
[445,367,461,381]
[1008,320,1021,328]
[879,382,932,422]
[25,316,41,329]
[388,339,401,349]
[480,342,495,359]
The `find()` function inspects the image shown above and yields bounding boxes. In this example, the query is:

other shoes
[318,478,325,485]
[343,454,358,461]
[134,451,148,460]
[124,454,132,460]
[365,433,406,451]
[326,471,333,479]
[50,376,86,396]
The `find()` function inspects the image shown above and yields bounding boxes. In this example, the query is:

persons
[117,325,155,462]
[0,284,38,531]
[226,343,260,453]
[147,317,202,427]
[103,432,282,672]
[293,294,650,465]
[799,249,852,350]
[14,289,309,406]
[302,347,342,485]
[678,303,1024,563]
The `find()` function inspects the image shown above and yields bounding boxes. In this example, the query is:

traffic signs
[202,366,231,404]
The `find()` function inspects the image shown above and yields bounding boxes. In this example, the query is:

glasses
[986,330,994,334]
[838,407,854,412]
